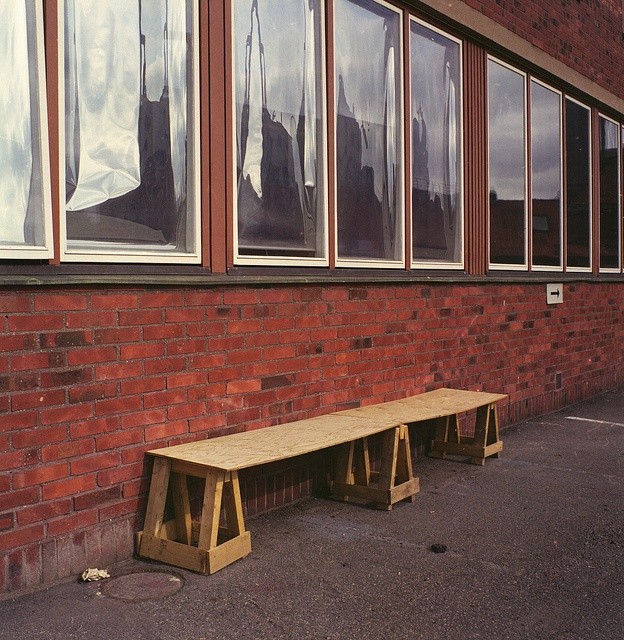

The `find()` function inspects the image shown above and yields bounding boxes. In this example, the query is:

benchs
[136,386,509,576]
